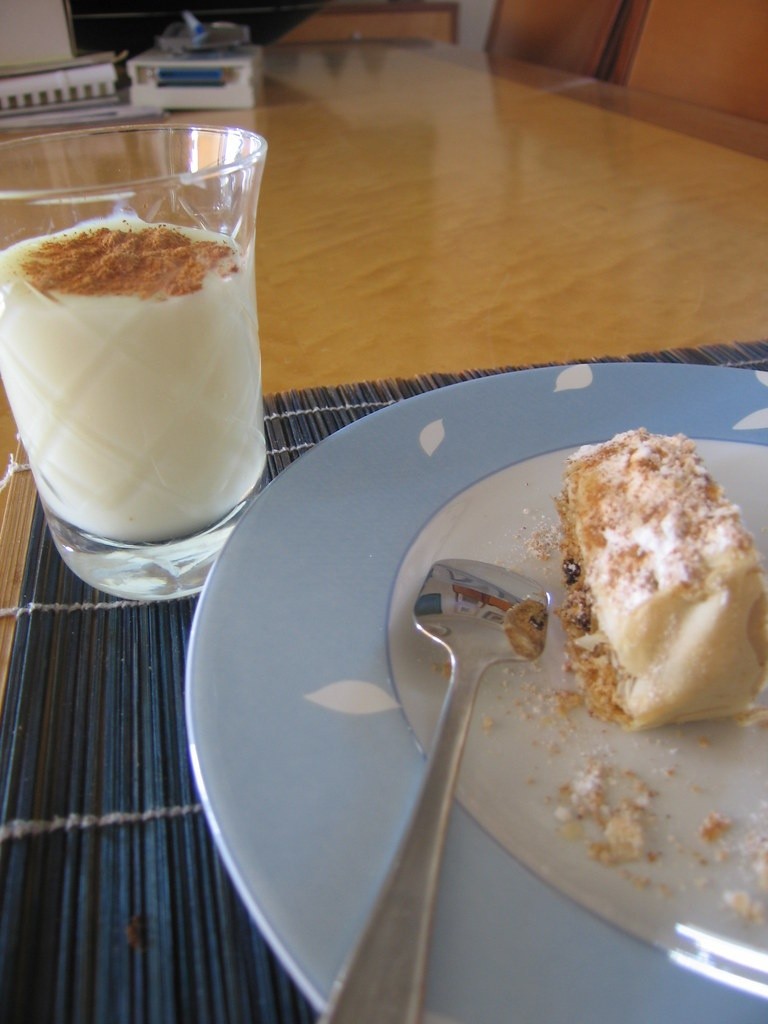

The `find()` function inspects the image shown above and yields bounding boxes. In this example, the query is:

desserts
[554,429,767,730]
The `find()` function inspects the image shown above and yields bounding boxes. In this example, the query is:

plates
[184,363,768,1024]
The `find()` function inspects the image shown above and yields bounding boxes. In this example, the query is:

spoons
[317,558,548,1024]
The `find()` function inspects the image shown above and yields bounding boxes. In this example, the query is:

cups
[1,122,273,603]
